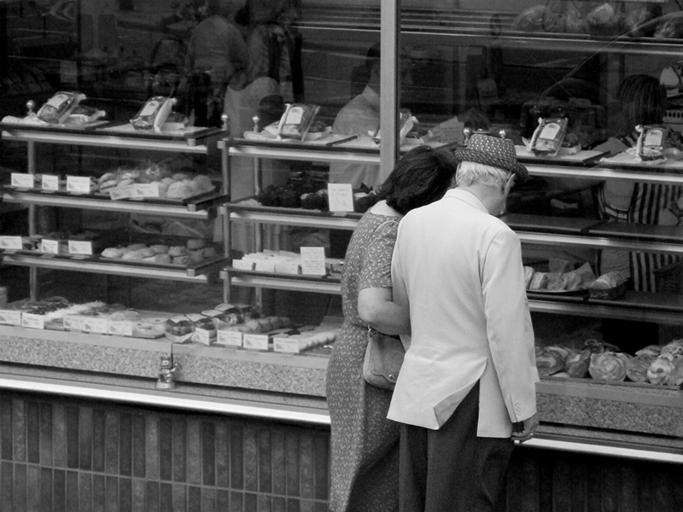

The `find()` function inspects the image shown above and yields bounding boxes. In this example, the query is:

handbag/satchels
[363,330,405,390]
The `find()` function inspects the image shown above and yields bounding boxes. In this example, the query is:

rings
[529,431,533,435]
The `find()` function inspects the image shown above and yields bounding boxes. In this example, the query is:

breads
[523,264,623,291]
[99,164,212,199]
[534,340,683,387]
[99,239,215,265]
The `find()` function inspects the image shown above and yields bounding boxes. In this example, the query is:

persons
[328,44,413,267]
[384,129,542,510]
[597,76,682,351]
[323,147,458,511]
[146,0,306,127]
[190,15,288,315]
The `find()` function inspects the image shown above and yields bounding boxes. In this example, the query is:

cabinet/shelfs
[2,110,228,304]
[217,116,682,347]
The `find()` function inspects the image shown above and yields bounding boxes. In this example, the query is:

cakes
[255,178,379,213]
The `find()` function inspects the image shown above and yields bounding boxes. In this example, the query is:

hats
[454,132,528,182]
